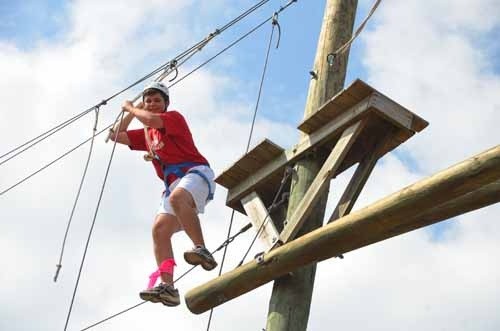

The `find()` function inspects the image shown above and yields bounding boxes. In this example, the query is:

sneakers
[184,247,217,271]
[139,283,180,306]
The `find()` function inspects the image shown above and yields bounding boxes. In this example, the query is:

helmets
[144,82,169,96]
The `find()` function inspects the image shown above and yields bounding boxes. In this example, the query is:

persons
[109,82,218,307]
